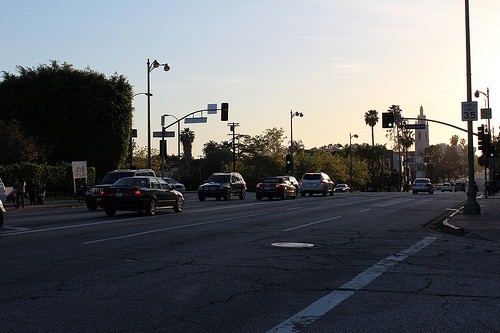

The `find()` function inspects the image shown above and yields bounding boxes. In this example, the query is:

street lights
[145,58,170,168]
[349,134,359,192]
[475,89,492,197]
[128,92,153,169]
[290,111,304,176]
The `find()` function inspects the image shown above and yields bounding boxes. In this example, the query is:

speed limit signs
[461,101,478,121]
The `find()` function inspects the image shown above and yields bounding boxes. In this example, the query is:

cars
[333,184,350,193]
[436,177,469,193]
[97,175,185,216]
[255,175,301,200]
[413,177,434,195]
[159,178,185,195]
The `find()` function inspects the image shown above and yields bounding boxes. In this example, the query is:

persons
[14,178,24,208]
[25,181,36,205]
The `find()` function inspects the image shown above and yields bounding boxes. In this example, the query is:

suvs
[84,168,156,213]
[198,172,247,202]
[299,173,335,196]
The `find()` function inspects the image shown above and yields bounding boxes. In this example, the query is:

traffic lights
[382,112,393,129]
[478,124,484,150]
[286,154,291,170]
[489,146,495,158]
